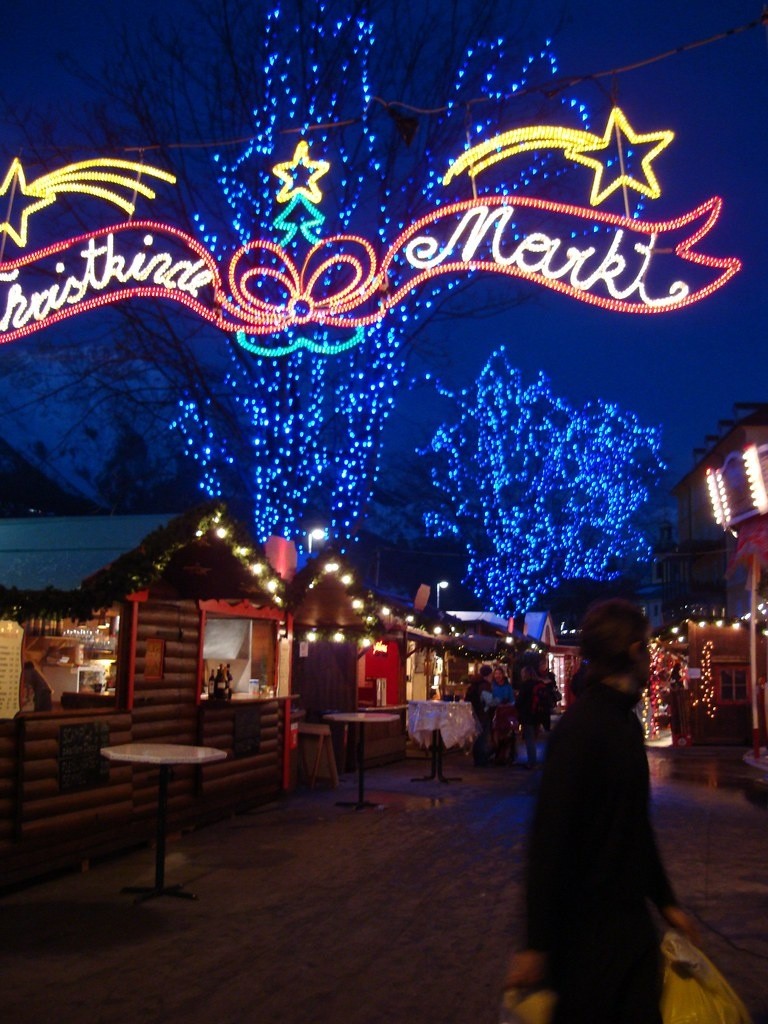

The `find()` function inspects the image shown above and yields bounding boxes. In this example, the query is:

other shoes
[525,763,536,770]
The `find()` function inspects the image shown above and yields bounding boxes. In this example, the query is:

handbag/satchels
[661,931,752,1023]
[551,686,562,701]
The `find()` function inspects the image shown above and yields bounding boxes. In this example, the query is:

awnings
[406,625,495,659]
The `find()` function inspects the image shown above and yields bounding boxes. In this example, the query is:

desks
[322,713,402,811]
[407,699,473,785]
[98,743,226,904]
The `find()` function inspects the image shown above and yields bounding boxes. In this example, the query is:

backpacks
[531,682,547,716]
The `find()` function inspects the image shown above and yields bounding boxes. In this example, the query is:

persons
[463,652,561,768]
[500,597,692,1024]
